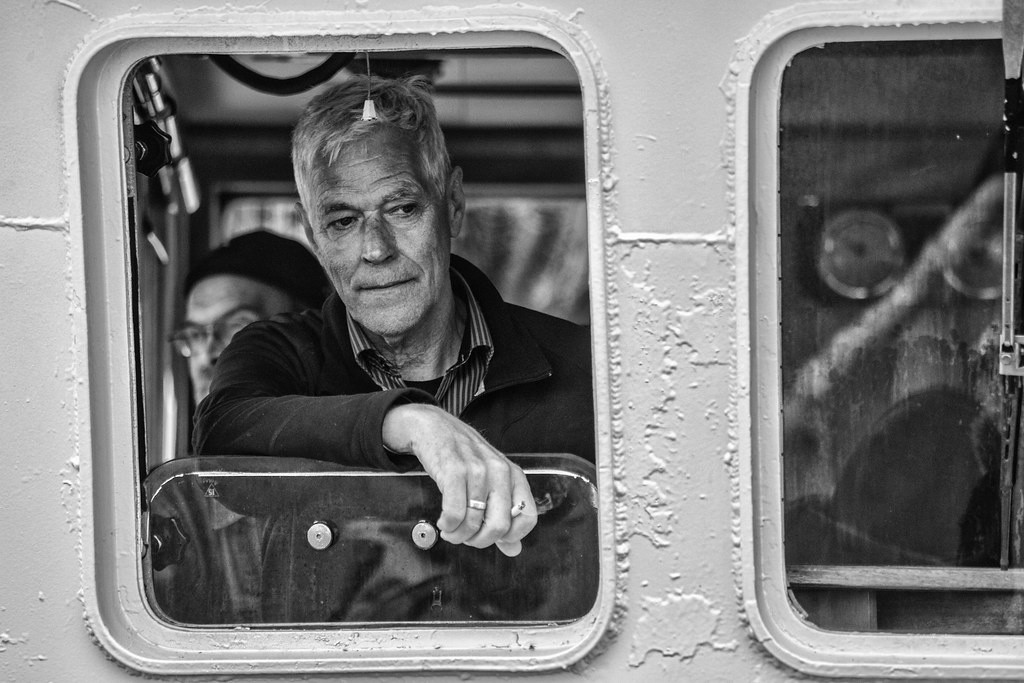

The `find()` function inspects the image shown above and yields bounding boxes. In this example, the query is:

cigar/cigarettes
[510,498,526,518]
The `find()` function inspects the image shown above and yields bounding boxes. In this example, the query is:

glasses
[167,310,251,358]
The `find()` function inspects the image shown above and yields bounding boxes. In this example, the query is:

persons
[182,76,598,628]
[151,228,331,625]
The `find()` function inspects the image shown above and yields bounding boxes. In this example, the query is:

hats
[181,230,333,308]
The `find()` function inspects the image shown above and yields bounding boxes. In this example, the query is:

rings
[467,499,486,510]
